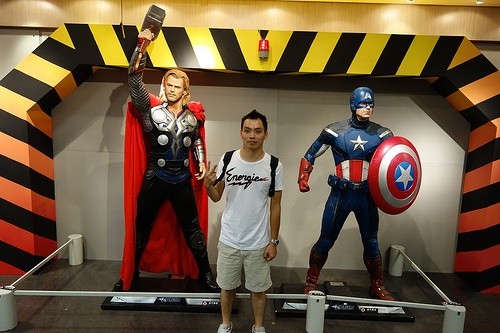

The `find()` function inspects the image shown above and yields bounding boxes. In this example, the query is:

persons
[113,29,221,293]
[204,109,283,333]
[298,87,394,301]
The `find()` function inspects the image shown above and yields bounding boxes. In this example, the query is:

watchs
[271,239,280,246]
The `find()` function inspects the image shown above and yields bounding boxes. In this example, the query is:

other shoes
[252,324,266,333]
[217,322,233,333]
